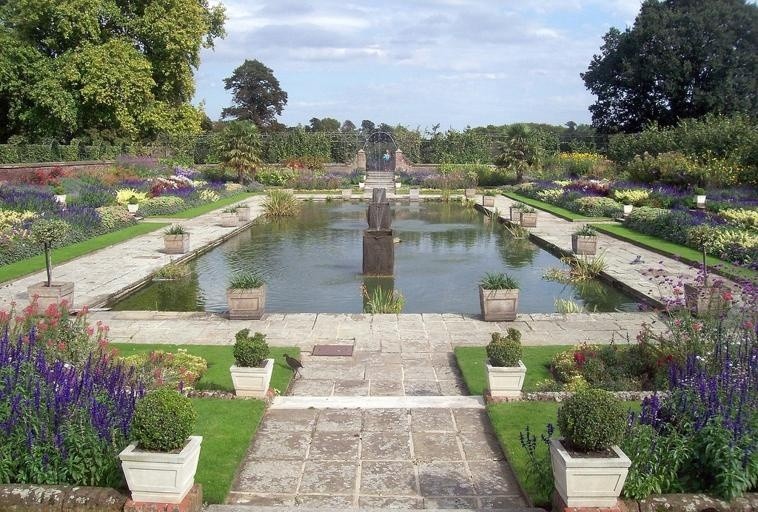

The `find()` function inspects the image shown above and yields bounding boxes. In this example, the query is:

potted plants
[483,191,496,207]
[549,389,631,508]
[118,388,203,504]
[571,224,599,255]
[27,218,74,315]
[486,337,527,396]
[220,208,239,227]
[226,271,266,320]
[474,271,522,321]
[509,202,525,221]
[229,337,274,396]
[684,223,731,318]
[520,208,538,227]
[232,203,250,220]
[162,225,190,254]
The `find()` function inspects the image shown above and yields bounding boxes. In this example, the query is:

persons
[382,149,391,171]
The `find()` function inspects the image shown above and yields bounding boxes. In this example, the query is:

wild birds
[282,353,304,379]
